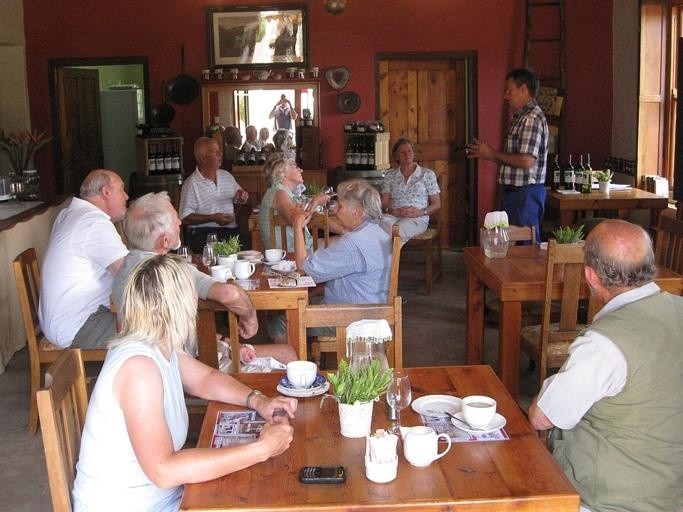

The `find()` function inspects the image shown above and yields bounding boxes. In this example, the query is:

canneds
[296,69,306,79]
[228,68,239,79]
[214,69,224,80]
[200,70,209,81]
[307,66,320,79]
[286,68,297,79]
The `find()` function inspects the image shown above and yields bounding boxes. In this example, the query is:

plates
[277,377,330,397]
[410,393,461,418]
[450,413,506,433]
[271,264,296,272]
[237,250,262,259]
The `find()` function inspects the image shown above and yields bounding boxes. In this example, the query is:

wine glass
[387,368,412,434]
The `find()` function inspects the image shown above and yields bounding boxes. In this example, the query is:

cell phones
[298,466,346,483]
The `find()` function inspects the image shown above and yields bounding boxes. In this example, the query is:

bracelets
[243,387,262,412]
[423,207,428,218]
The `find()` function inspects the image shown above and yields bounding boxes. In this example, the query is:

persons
[258,155,330,256]
[465,66,549,247]
[211,93,299,156]
[178,138,250,253]
[36,169,299,374]
[381,139,442,251]
[257,178,393,344]
[526,217,683,512]
[73,252,300,511]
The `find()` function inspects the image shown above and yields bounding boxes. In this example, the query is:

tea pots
[398,425,453,468]
[346,324,393,397]
[253,69,275,80]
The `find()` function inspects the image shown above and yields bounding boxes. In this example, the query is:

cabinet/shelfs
[345,131,391,171]
[144,136,185,176]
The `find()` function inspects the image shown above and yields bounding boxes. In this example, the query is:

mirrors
[201,81,321,171]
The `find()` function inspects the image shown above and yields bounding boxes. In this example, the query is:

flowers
[0,126,55,180]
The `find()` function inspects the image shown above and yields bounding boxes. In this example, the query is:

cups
[365,431,398,484]
[285,360,317,387]
[461,395,498,426]
[178,232,296,282]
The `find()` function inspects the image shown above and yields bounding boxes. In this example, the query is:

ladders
[524,0,565,186]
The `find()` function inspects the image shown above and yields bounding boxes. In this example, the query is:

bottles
[551,153,591,194]
[237,145,266,165]
[200,67,240,80]
[288,66,320,79]
[22,169,41,201]
[147,142,179,174]
[344,136,375,169]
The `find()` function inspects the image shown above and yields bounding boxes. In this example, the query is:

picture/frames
[205,3,311,70]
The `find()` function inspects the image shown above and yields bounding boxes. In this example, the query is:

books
[535,85,564,157]
[642,174,671,202]
